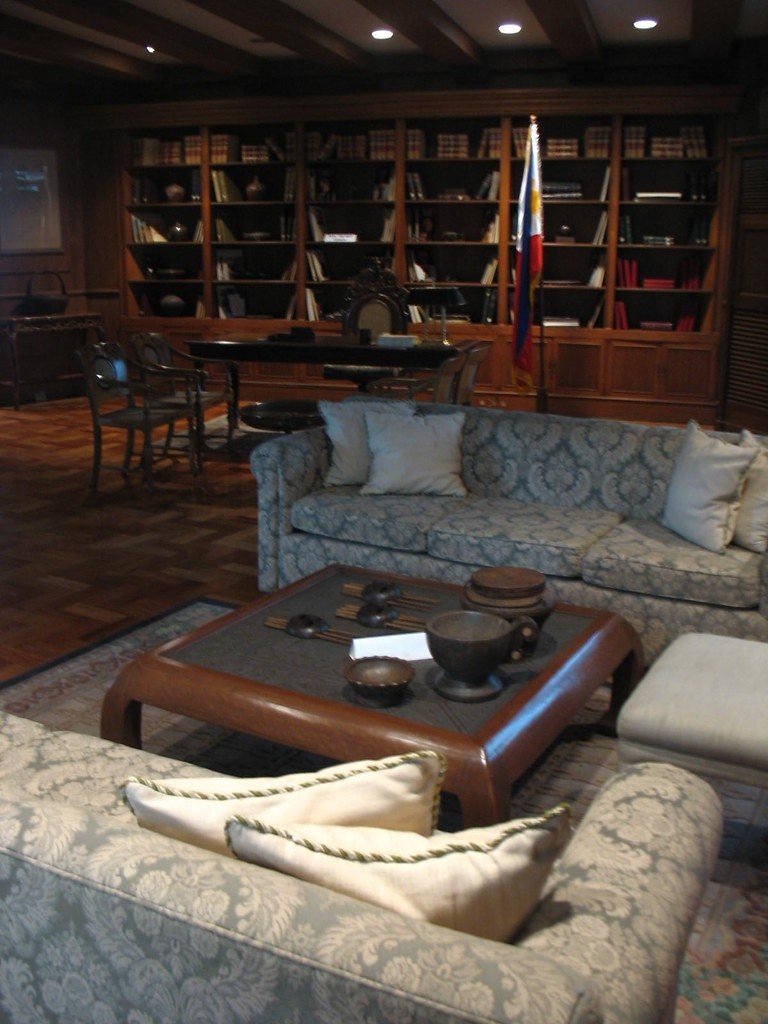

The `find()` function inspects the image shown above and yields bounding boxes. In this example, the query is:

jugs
[425,611,539,700]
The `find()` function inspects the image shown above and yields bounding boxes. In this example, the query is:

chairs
[433,341,494,406]
[68,324,204,499]
[322,256,410,397]
[129,324,243,464]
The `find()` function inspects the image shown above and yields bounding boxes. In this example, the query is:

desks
[181,335,480,456]
[0,312,104,412]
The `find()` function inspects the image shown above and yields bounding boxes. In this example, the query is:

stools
[615,633,768,788]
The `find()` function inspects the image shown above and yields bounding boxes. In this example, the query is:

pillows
[118,748,447,861]
[359,411,469,497]
[737,429,768,553]
[317,398,418,488]
[222,804,572,943]
[660,418,759,556]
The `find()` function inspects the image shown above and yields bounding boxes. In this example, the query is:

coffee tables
[99,564,644,832]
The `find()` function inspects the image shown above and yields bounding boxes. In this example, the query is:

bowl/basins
[345,656,416,703]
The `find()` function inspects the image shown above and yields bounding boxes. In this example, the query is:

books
[129,212,168,243]
[215,248,234,280]
[282,253,297,281]
[210,134,296,164]
[514,125,610,156]
[194,219,203,243]
[625,125,708,158]
[280,212,296,240]
[216,285,236,318]
[307,130,397,160]
[283,294,297,321]
[216,212,235,240]
[407,129,503,158]
[211,169,238,201]
[195,298,206,318]
[306,169,707,328]
[132,135,204,165]
[284,166,297,200]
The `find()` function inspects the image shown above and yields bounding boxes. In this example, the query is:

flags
[510,124,540,390]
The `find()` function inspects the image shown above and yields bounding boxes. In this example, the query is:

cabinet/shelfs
[52,87,752,425]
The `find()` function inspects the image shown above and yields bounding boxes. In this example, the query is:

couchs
[0,710,723,1024]
[249,403,768,666]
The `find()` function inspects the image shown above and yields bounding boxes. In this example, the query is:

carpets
[1,596,768,1024]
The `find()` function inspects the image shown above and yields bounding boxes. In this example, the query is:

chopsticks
[264,578,436,645]
